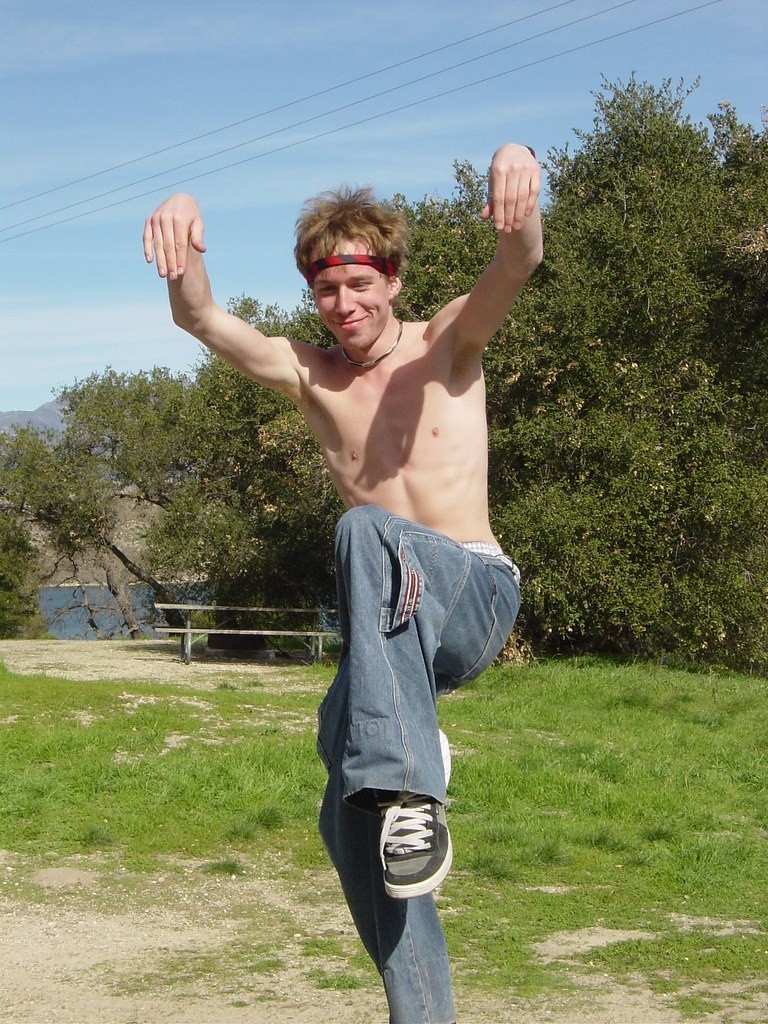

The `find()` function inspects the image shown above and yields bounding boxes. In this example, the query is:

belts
[466,542,520,579]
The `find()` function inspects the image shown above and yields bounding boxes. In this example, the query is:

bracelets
[524,145,535,159]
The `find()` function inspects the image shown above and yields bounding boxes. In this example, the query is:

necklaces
[342,319,403,367]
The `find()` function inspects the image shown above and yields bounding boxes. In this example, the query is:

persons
[142,126,542,1024]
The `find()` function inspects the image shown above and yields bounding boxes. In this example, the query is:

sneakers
[377,789,452,898]
